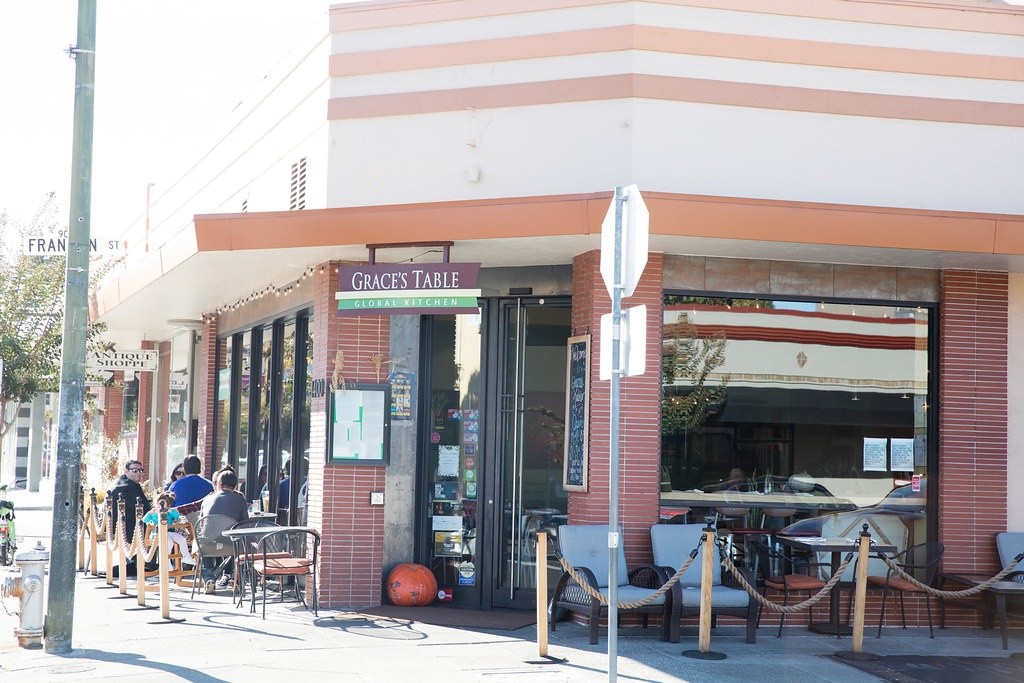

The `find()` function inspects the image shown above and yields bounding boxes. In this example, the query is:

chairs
[644,519,759,644]
[550,525,671,646]
[849,541,945,639]
[990,533,1024,628]
[750,539,840,639]
[106,493,320,621]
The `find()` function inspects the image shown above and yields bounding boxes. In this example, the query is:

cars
[667,476,857,531]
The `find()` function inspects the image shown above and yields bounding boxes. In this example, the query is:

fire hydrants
[1,541,49,647]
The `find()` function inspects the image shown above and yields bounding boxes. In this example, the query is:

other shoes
[205,579,215,593]
[218,574,230,589]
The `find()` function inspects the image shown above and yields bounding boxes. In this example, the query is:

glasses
[129,467,144,474]
[174,471,185,475]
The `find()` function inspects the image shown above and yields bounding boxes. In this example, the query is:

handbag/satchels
[661,465,672,491]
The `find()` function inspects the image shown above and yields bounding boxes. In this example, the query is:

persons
[110,460,158,576]
[141,454,248,594]
[258,459,307,525]
[199,470,248,594]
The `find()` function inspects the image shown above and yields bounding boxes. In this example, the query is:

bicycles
[0,501,14,565]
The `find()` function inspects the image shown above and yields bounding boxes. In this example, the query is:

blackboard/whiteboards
[563,333,590,492]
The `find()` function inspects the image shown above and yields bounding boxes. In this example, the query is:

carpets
[358,604,537,632]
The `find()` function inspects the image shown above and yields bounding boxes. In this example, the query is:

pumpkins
[386,563,438,607]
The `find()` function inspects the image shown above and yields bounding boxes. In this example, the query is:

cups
[253,500,261,515]
[261,490,269,512]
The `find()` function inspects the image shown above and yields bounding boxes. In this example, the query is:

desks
[221,526,314,609]
[937,574,1024,649]
[778,536,897,635]
